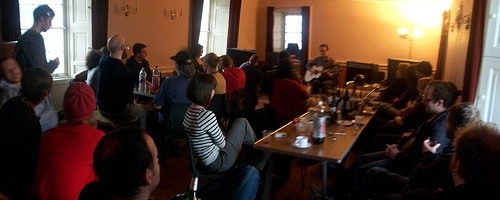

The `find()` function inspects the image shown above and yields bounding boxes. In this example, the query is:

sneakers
[311,185,333,199]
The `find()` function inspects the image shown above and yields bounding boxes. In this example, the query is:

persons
[14,5,60,96]
[1,36,500,200]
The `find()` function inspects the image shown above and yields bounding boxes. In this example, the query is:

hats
[64,82,96,121]
[170,51,193,64]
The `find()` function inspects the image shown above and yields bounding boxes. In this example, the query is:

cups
[295,136,308,147]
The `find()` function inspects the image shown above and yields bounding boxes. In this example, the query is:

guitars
[304,65,336,82]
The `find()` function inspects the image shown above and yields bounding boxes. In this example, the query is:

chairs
[158,120,225,200]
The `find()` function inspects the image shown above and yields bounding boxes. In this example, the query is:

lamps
[397,26,424,42]
[122,1,140,18]
[167,7,183,23]
[443,2,471,33]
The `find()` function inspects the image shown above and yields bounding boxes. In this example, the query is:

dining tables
[251,95,380,200]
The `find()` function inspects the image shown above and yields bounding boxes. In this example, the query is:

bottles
[152,66,160,94]
[183,174,200,200]
[311,101,327,144]
[139,68,147,93]
[328,84,357,124]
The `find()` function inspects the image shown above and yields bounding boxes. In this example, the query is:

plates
[291,142,311,148]
